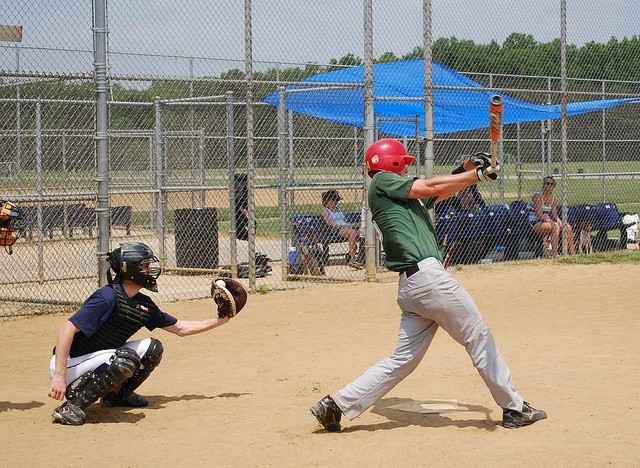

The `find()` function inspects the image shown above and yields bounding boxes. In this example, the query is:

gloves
[470,151,499,167]
[476,163,501,182]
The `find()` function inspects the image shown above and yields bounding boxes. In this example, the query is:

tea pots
[492,244,507,270]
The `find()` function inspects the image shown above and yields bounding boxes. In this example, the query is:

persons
[436,196,460,219]
[320,189,363,268]
[528,177,576,256]
[310,139,547,433]
[49,241,247,424]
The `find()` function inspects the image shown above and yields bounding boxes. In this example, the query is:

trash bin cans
[174,208,219,275]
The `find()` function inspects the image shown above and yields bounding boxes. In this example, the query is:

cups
[477,258,492,270]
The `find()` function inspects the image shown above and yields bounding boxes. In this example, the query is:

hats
[324,189,344,201]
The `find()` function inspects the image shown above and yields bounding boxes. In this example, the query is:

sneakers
[51,399,86,425]
[502,402,547,428]
[310,393,344,433]
[101,389,149,407]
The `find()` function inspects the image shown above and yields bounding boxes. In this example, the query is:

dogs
[578,221,593,254]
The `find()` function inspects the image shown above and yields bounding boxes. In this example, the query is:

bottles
[288,247,296,266]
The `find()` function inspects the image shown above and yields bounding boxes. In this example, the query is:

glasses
[546,180,555,186]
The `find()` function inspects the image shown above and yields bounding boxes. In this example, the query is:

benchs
[19,206,47,241]
[49,204,85,239]
[289,212,365,274]
[86,206,132,235]
[436,204,520,264]
[510,200,636,258]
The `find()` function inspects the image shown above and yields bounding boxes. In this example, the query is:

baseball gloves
[211,277,248,319]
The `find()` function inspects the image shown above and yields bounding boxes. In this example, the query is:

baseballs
[216,279,226,288]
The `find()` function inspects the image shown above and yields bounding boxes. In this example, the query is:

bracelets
[452,163,464,172]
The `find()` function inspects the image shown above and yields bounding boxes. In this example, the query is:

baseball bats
[489,94,503,168]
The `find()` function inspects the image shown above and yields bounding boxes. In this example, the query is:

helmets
[105,239,161,294]
[365,138,417,177]
